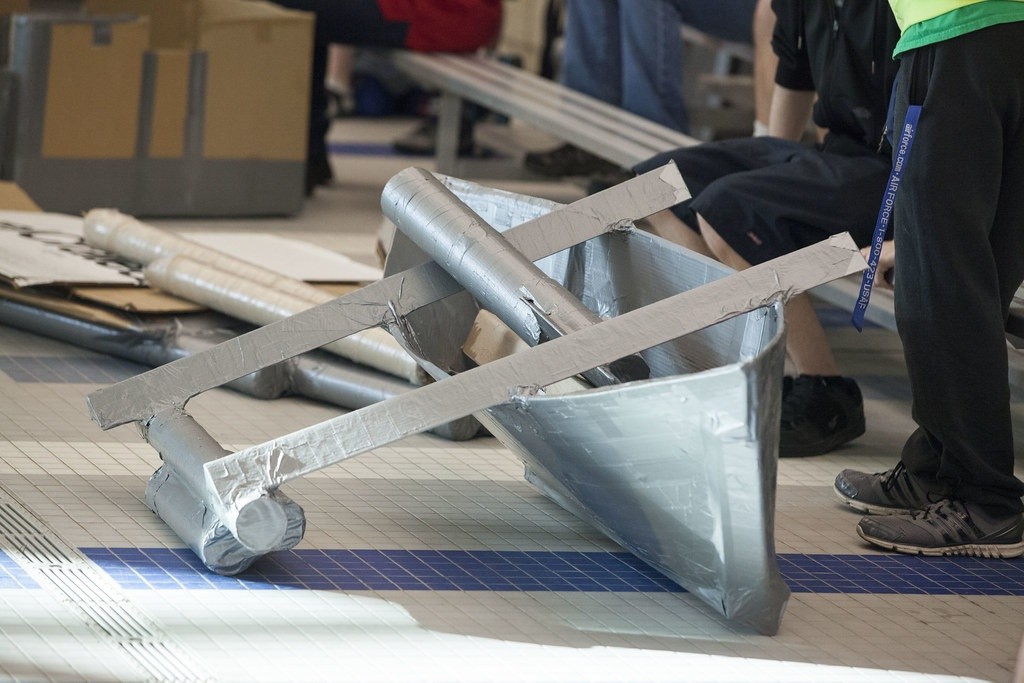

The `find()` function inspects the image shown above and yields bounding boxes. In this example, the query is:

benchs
[390,51,1023,370]
[678,24,757,138]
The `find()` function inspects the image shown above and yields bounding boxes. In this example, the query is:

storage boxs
[0,0,316,220]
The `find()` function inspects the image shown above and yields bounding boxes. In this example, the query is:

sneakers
[778,374,865,457]
[833,461,947,515]
[856,499,1024,559]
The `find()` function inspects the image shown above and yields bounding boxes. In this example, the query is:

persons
[272,0,507,181]
[525,0,756,194]
[630,0,902,457]
[835,0,1024,558]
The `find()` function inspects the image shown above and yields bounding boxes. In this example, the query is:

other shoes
[525,144,622,176]
[395,116,474,154]
[589,180,616,197]
